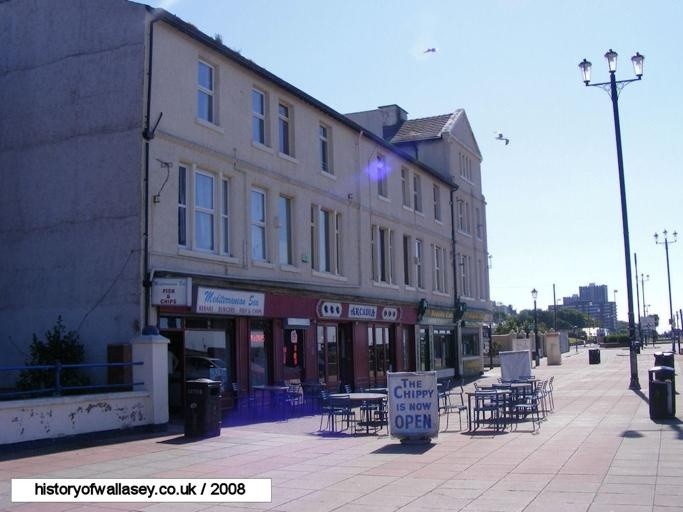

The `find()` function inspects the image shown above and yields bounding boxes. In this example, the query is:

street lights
[654,229,678,353]
[578,49,644,390]
[641,274,651,346]
[531,289,539,366]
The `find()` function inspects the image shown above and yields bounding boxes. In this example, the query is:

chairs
[232,374,555,437]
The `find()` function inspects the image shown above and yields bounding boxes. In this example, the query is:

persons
[168,351,179,382]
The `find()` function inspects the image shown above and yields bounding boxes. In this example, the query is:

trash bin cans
[185,378,222,438]
[589,349,600,364]
[649,351,675,418]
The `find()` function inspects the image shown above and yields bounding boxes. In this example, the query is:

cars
[173,355,232,392]
[249,362,266,387]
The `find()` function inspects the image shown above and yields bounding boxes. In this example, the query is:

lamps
[153,150,174,203]
